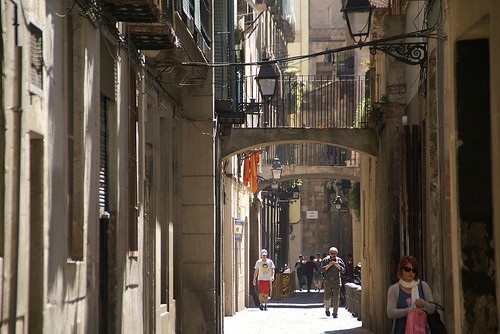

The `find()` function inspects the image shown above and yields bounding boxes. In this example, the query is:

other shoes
[326,310,330,316]
[332,312,338,318]
[259,303,263,311]
[264,305,267,310]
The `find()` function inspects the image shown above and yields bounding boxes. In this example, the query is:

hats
[260,249,267,256]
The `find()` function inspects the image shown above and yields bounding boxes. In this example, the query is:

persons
[347,253,353,282]
[386,254,436,334]
[295,254,305,291]
[251,249,277,311]
[312,253,323,291]
[320,246,347,318]
[302,256,319,293]
[353,260,364,285]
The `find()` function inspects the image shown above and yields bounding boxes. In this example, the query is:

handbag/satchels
[404,304,426,334]
[422,302,448,334]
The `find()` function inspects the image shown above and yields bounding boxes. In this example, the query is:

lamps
[333,196,344,210]
[255,154,284,186]
[292,183,300,203]
[254,58,280,109]
[339,0,429,66]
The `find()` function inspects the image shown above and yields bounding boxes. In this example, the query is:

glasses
[403,266,416,272]
